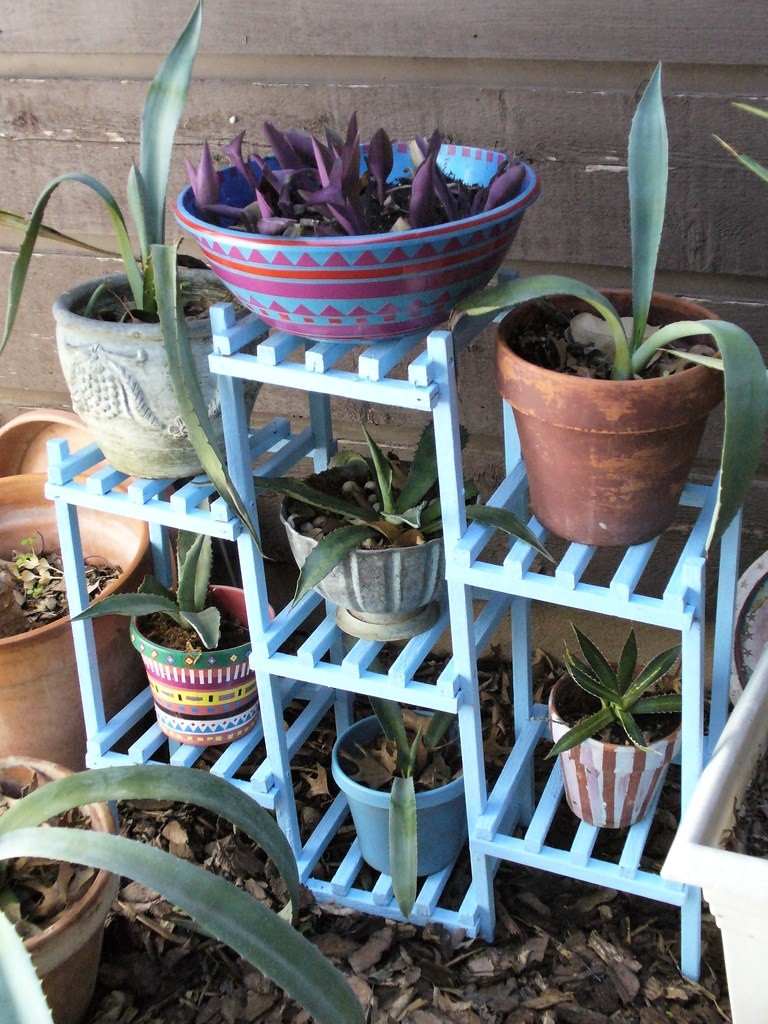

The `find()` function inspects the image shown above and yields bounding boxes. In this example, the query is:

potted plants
[332,633,469,919]
[62,475,260,747]
[1,1,278,562]
[440,59,767,558]
[0,754,366,1024]
[542,618,680,830]
[171,110,542,345]
[252,411,558,645]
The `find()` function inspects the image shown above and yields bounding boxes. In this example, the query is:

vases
[659,640,768,1024]
[1,470,154,798]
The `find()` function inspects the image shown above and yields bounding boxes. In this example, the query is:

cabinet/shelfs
[42,269,741,985]
